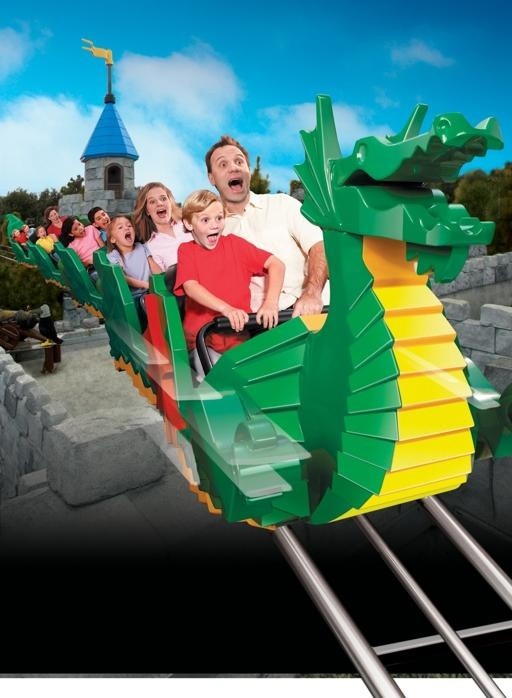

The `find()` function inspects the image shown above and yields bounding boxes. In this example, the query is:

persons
[11,206,111,285]
[205,134,330,336]
[172,190,286,377]
[135,181,194,272]
[107,215,163,336]
